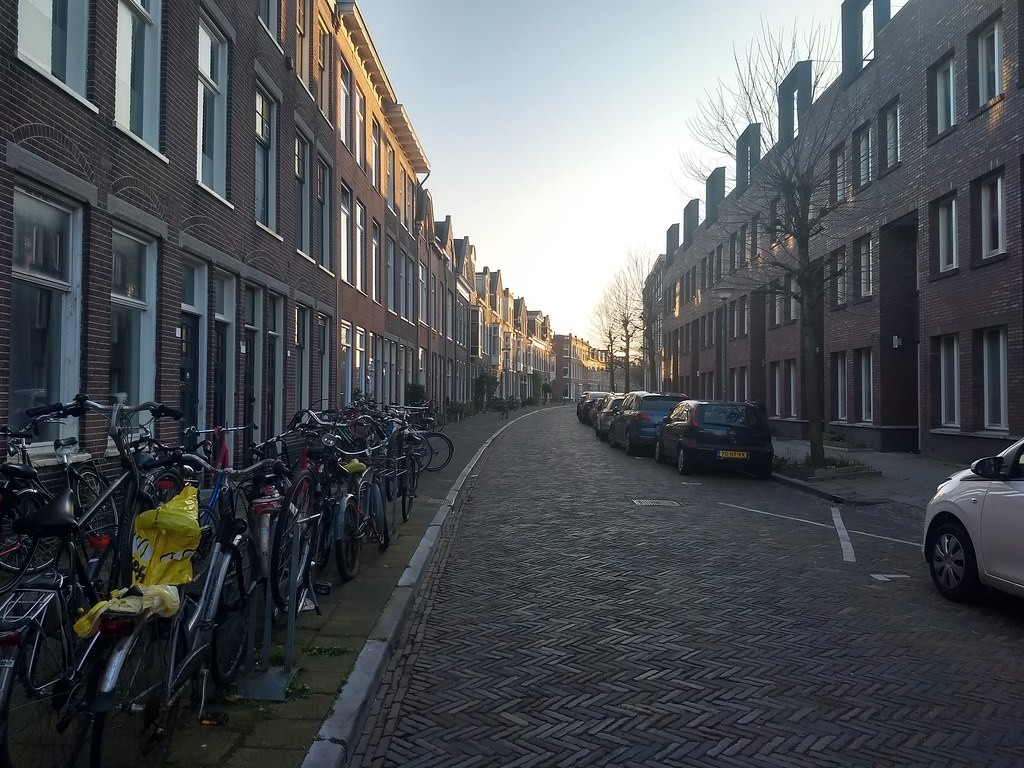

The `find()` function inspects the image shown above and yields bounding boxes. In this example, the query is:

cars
[920,436,1024,603]
[576,391,629,441]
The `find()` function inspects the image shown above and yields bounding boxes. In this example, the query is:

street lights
[715,284,736,398]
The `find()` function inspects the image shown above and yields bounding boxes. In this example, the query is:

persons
[498,396,509,420]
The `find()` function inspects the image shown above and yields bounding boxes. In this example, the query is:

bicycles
[494,394,528,412]
[0,377,454,767]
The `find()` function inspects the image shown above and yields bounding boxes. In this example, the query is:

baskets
[267,433,305,466]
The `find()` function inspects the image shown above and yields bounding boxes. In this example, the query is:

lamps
[893,334,903,349]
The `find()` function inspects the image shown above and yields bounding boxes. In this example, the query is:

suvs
[607,390,690,456]
[654,399,774,479]
